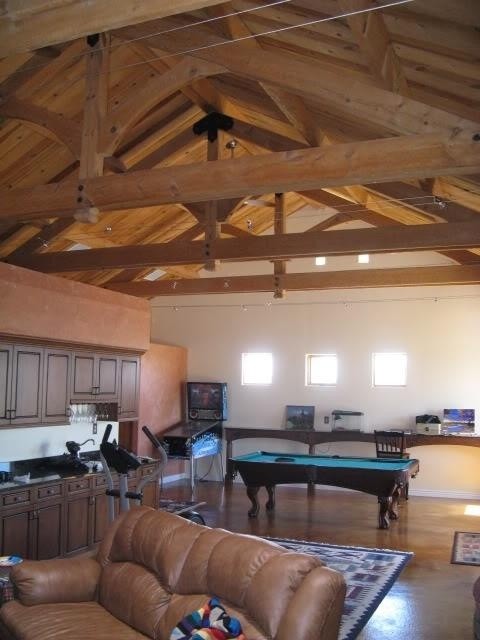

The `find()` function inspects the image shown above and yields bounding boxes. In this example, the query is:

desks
[224,427,480,498]
[228,450,419,529]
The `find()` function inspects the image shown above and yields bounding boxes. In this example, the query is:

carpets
[450,531,480,566]
[261,535,414,640]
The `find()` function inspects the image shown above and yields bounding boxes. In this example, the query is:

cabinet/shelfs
[63,474,119,558]
[0,343,44,428]
[118,356,141,422]
[125,465,159,511]
[70,350,119,404]
[1,482,65,562]
[41,347,71,426]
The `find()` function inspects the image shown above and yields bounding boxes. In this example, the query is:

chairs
[374,430,410,500]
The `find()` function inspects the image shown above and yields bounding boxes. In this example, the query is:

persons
[199,390,211,411]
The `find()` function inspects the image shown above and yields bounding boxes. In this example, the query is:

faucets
[68,439,95,451]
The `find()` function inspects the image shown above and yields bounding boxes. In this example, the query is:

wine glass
[69,403,108,422]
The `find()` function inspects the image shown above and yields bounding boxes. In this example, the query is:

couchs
[1,505,346,639]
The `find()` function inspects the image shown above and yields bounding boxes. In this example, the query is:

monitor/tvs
[186,382,227,420]
